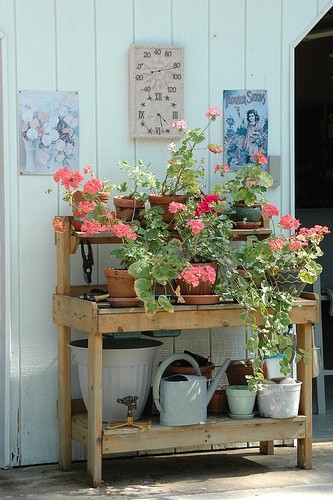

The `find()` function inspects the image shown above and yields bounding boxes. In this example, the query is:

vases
[225,384,258,416]
[228,267,264,301]
[104,266,139,298]
[148,194,188,222]
[228,202,263,223]
[265,268,308,301]
[70,190,109,232]
[222,359,259,386]
[112,193,148,224]
[174,262,218,295]
[66,336,164,421]
[170,359,215,388]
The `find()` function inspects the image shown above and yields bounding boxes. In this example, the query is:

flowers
[44,108,331,396]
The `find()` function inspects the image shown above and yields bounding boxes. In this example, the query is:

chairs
[295,209,333,414]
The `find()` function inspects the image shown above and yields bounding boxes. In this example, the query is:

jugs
[152,352,231,426]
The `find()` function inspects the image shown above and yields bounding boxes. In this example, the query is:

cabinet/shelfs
[51,214,321,489]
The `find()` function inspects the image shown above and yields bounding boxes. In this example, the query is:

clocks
[129,44,185,139]
